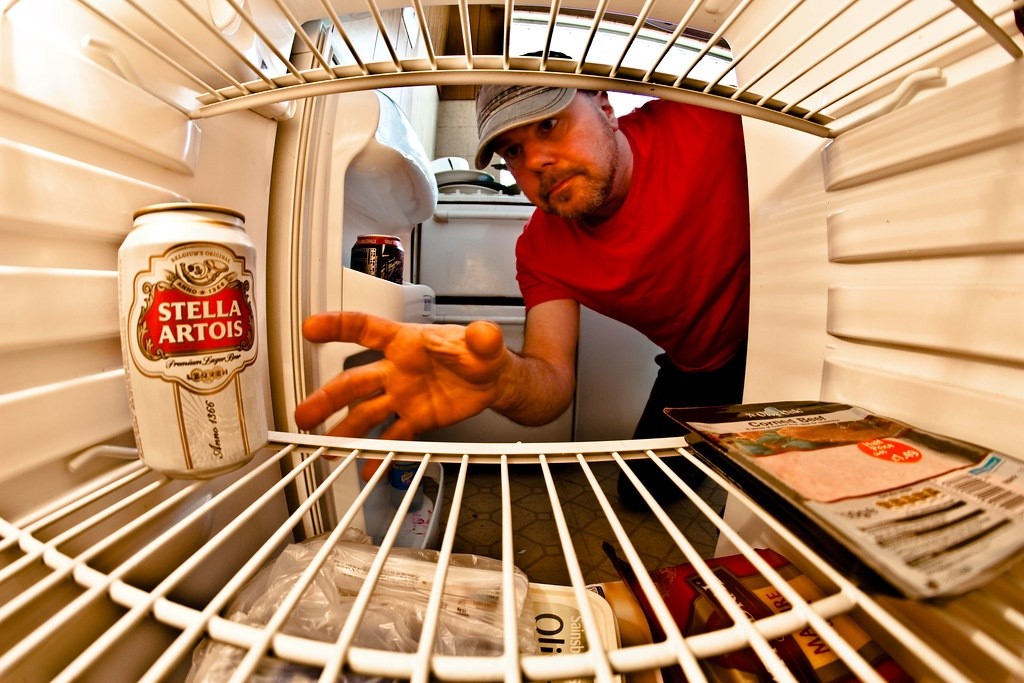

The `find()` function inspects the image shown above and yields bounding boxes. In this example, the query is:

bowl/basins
[430,156,470,173]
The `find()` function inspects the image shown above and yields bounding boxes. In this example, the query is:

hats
[474,86,577,170]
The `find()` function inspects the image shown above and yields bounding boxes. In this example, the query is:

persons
[290,50,750,513]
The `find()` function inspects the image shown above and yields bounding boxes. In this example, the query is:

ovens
[412,193,574,453]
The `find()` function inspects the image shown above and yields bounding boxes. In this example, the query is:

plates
[433,170,501,195]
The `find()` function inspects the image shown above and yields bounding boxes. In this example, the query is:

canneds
[118,201,269,480]
[349,234,404,285]
